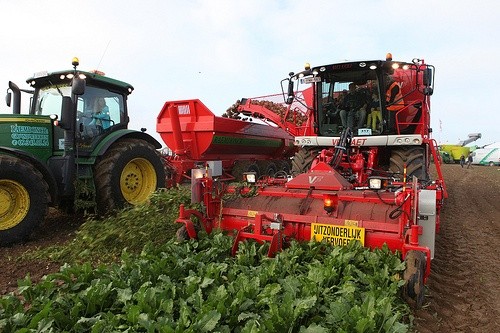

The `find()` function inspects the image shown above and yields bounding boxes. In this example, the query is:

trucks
[465,148,500,167]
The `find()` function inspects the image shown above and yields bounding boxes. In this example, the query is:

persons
[466,154,473,169]
[340,83,367,135]
[460,155,465,168]
[367,93,380,130]
[89,95,110,129]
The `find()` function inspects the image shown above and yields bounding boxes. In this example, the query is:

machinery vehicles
[154,52,448,311]
[438,132,482,163]
[0,57,168,247]
